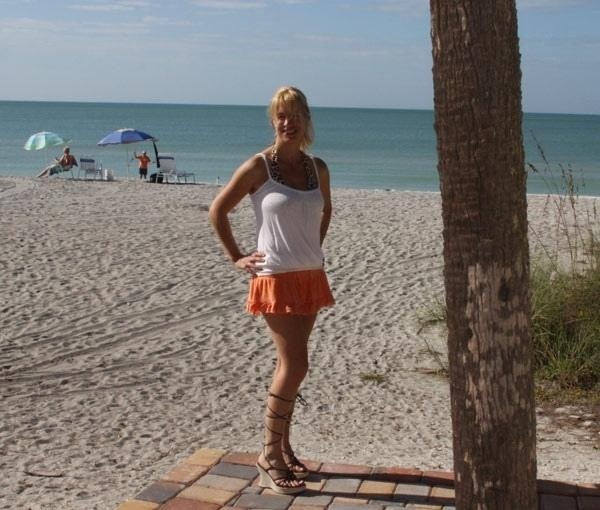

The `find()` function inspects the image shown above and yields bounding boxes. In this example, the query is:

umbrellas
[23,131,64,164]
[97,127,154,180]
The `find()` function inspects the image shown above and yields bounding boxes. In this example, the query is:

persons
[133,150,150,180]
[207,85,336,495]
[36,147,78,178]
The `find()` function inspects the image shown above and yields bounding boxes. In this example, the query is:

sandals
[281,445,310,479]
[256,454,306,494]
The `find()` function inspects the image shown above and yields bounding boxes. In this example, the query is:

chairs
[48,158,104,182]
[155,156,196,185]
